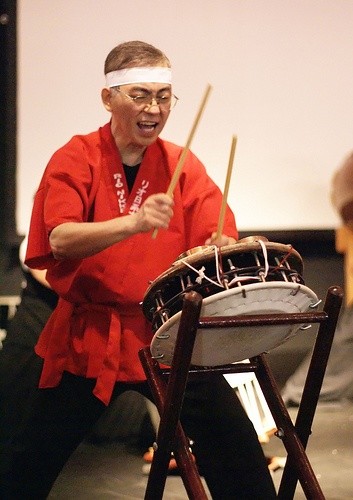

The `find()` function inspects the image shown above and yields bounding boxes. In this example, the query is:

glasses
[110,86,180,111]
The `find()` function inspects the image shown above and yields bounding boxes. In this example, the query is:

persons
[0,37,282,500]
[0,266,198,474]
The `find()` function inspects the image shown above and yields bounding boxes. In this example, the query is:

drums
[139,234,317,368]
